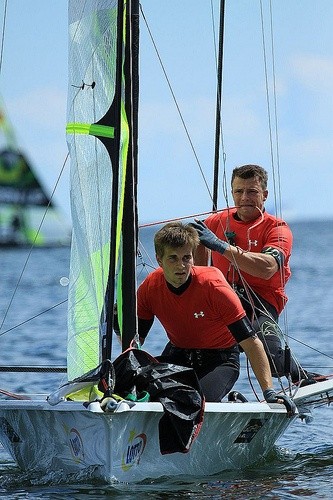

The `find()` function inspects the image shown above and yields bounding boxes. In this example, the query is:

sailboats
[0,100,69,249]
[0,0,333,486]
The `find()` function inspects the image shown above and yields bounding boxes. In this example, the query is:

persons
[192,164,328,388]
[111,221,300,416]
